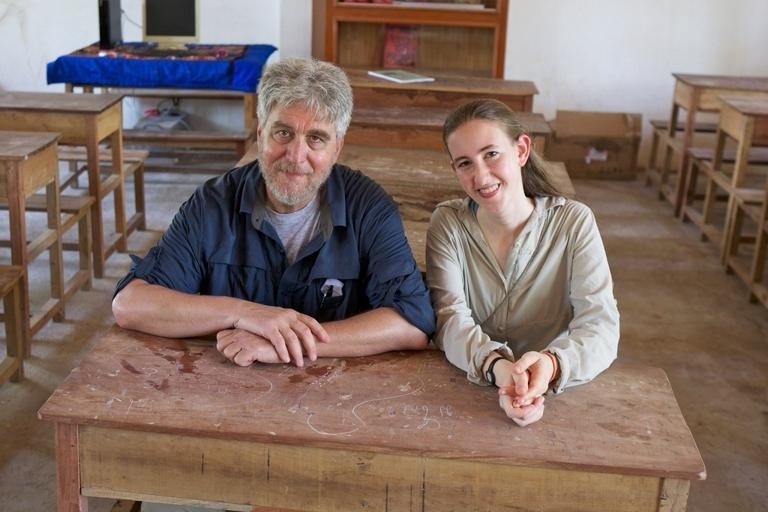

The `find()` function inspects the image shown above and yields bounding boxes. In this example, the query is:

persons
[426,99,622,425]
[112,59,436,366]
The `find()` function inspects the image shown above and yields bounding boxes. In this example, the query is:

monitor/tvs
[143,0,199,52]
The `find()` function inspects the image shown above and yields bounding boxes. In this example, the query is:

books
[383,25,418,69]
[367,67,435,86]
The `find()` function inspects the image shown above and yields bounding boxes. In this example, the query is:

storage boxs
[546,107,642,184]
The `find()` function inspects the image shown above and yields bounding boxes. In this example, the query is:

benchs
[57,144,148,238]
[1,193,94,292]
[0,265,24,388]
[647,118,718,187]
[683,148,766,226]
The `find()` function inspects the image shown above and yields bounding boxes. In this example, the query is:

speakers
[99,0,122,48]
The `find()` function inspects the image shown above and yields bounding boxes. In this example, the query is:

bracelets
[543,350,559,382]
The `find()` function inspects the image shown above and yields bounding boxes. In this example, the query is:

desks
[698,95,766,262]
[1,130,64,349]
[660,68,767,216]
[37,321,707,512]
[56,39,265,181]
[1,91,127,278]
[333,66,538,116]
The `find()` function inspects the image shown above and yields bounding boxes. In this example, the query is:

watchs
[485,357,504,386]
